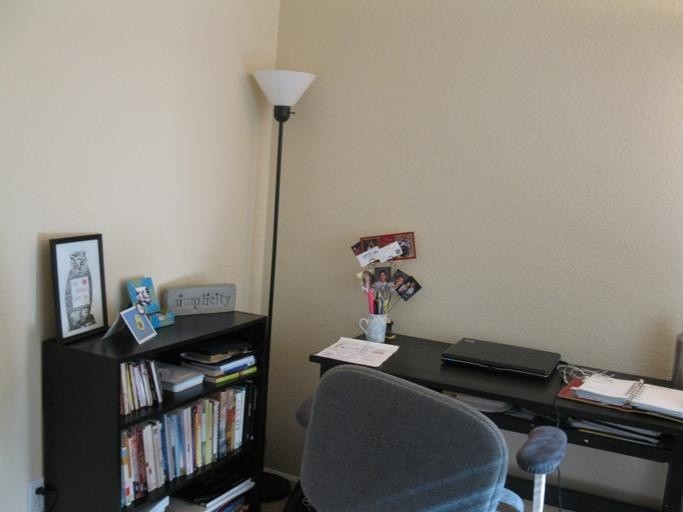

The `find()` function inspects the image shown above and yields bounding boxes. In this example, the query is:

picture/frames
[125,276,160,315]
[49,233,109,345]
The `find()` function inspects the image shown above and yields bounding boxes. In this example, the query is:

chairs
[283,365,568,512]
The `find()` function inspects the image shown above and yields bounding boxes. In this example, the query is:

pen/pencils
[361,286,401,314]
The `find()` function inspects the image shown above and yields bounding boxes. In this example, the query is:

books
[116,342,256,512]
[442,373,683,449]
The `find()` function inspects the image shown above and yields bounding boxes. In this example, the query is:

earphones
[563,370,569,384]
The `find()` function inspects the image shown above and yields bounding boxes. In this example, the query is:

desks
[310,330,683,512]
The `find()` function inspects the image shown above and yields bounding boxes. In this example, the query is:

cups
[359,313,388,343]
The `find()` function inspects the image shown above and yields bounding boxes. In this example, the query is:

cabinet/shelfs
[41,311,271,512]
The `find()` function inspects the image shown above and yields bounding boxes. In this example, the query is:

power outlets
[26,476,44,512]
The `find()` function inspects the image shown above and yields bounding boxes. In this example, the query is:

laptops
[440,338,562,378]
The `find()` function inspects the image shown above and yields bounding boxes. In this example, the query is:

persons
[352,236,416,303]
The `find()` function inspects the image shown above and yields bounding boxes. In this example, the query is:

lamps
[248,69,316,504]
